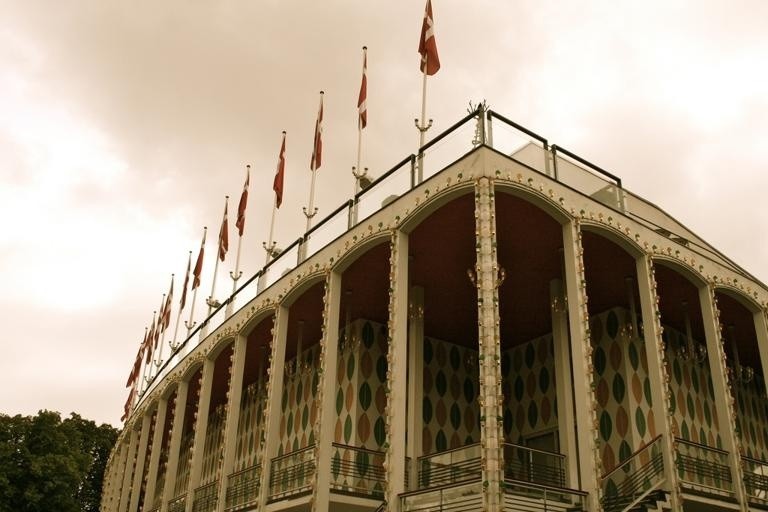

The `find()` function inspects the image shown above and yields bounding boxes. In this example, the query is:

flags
[237,168,249,235]
[121,252,191,421]
[218,198,228,260]
[357,49,368,128]
[419,0,440,76]
[311,99,322,169]
[273,132,286,208]
[193,229,205,290]
